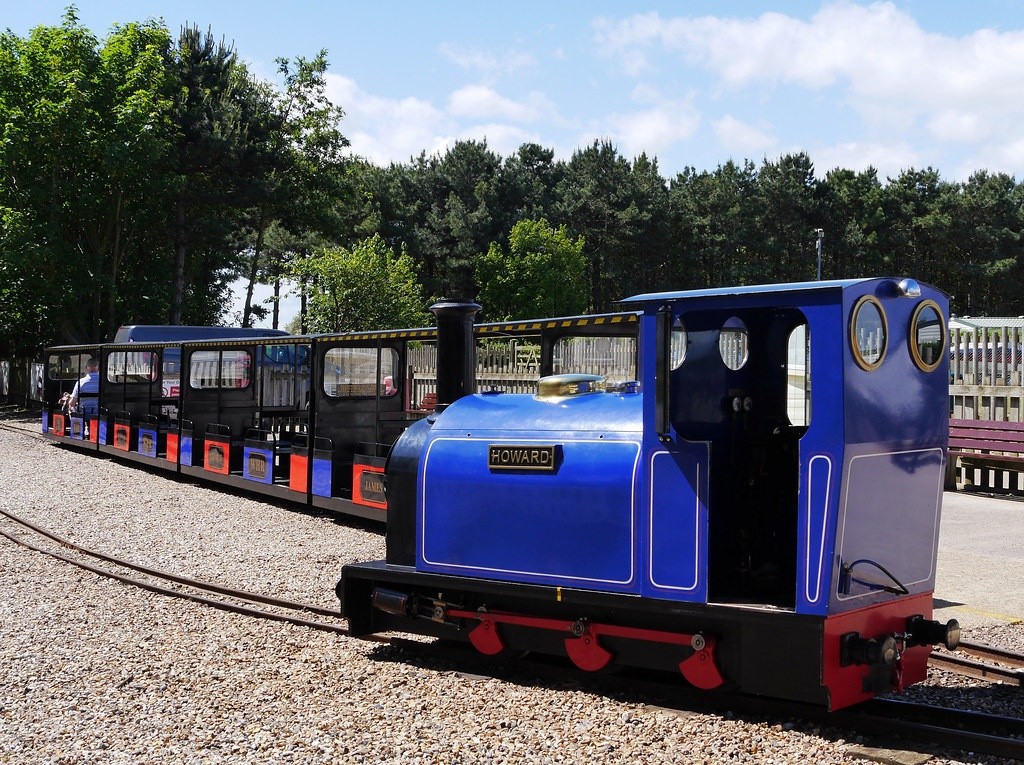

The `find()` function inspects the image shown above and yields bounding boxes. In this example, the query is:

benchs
[407,392,437,414]
[108,417,354,498]
[943,418,1024,490]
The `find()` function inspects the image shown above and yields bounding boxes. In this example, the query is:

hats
[87,358,100,366]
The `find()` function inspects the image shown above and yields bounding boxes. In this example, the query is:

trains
[42,338,636,529]
[334,275,962,715]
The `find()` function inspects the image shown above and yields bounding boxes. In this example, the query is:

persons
[68,358,99,435]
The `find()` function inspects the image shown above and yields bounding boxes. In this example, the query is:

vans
[112,326,346,410]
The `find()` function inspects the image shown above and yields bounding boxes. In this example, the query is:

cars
[948,343,1023,384]
